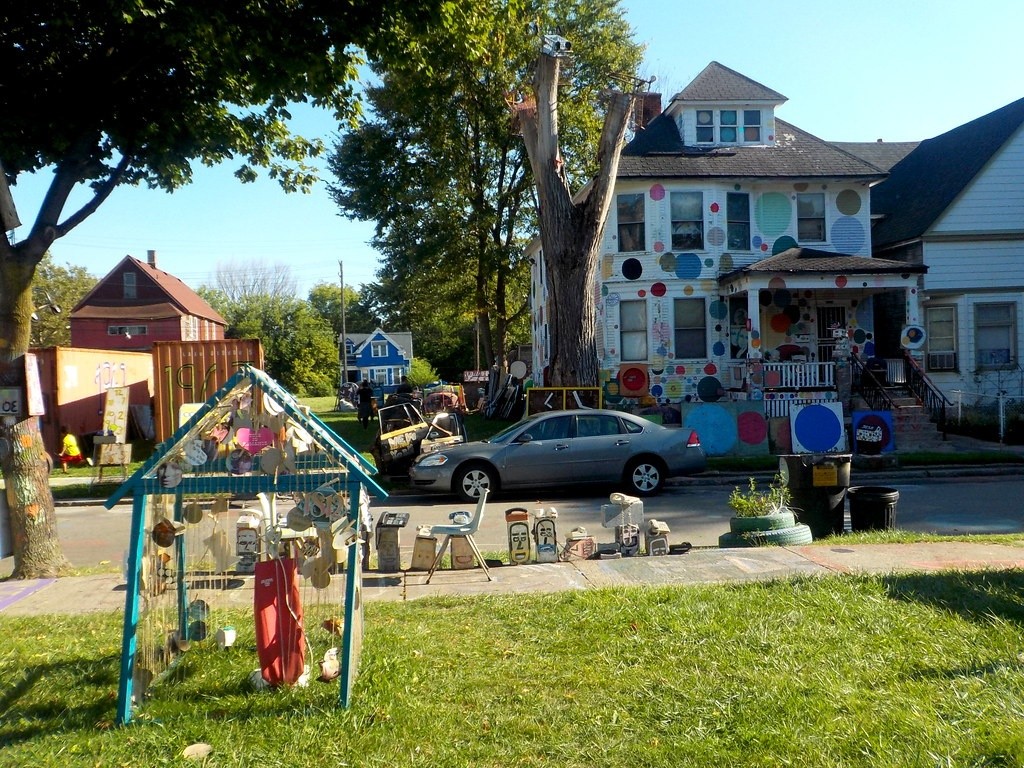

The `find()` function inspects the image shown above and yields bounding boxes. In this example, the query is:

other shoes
[371,420,374,422]
[62,472,67,475]
[87,458,94,466]
[359,422,361,424]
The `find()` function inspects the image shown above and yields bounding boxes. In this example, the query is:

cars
[411,409,708,503]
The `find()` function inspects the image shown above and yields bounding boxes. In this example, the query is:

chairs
[864,357,887,386]
[426,488,491,584]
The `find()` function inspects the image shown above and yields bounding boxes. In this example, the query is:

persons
[355,379,373,429]
[411,386,424,424]
[51,422,94,474]
[396,376,413,398]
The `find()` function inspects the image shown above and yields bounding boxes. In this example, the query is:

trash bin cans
[843,484,900,534]
[775,450,852,541]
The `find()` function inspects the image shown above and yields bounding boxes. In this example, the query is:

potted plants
[719,472,813,548]
